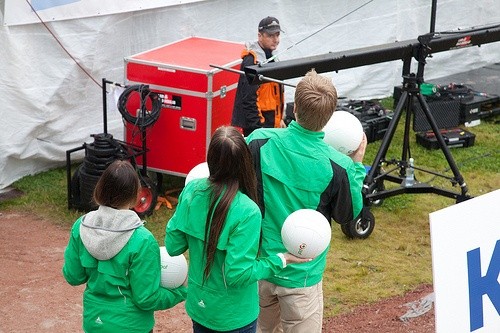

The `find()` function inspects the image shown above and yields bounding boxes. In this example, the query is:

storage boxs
[415,100,476,150]
[125,36,245,178]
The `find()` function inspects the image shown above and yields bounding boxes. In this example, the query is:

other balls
[158,246,188,289]
[322,110,364,156]
[282,208,332,260]
[185,161,209,188]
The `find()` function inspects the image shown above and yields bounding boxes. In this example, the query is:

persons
[245,74,367,333]
[62,160,187,333]
[164,125,314,333]
[230,17,285,137]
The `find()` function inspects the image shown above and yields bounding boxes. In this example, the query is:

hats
[258,16,285,35]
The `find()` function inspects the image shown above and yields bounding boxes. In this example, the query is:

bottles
[404,158,414,187]
